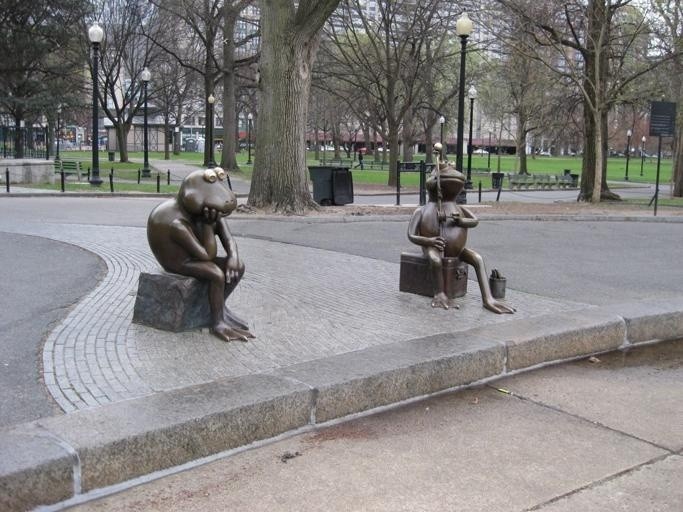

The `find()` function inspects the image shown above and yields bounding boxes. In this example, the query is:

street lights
[624,129,632,180]
[455,12,473,204]
[640,136,646,176]
[88,21,103,185]
[247,113,253,164]
[141,67,151,177]
[208,94,215,169]
[465,85,477,189]
[439,116,445,144]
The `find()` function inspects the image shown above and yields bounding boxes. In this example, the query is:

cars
[535,152,552,158]
[358,147,366,152]
[378,148,390,152]
[472,149,489,154]
[321,145,349,152]
[100,135,108,146]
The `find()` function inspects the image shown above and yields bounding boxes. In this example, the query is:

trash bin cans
[564,169,578,187]
[307,166,354,206]
[492,173,504,189]
[108,152,115,161]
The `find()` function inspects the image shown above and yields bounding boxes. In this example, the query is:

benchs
[54,160,87,181]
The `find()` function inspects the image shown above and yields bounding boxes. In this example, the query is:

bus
[182,125,224,151]
[66,125,85,148]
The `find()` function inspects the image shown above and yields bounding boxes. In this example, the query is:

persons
[354,150,364,171]
